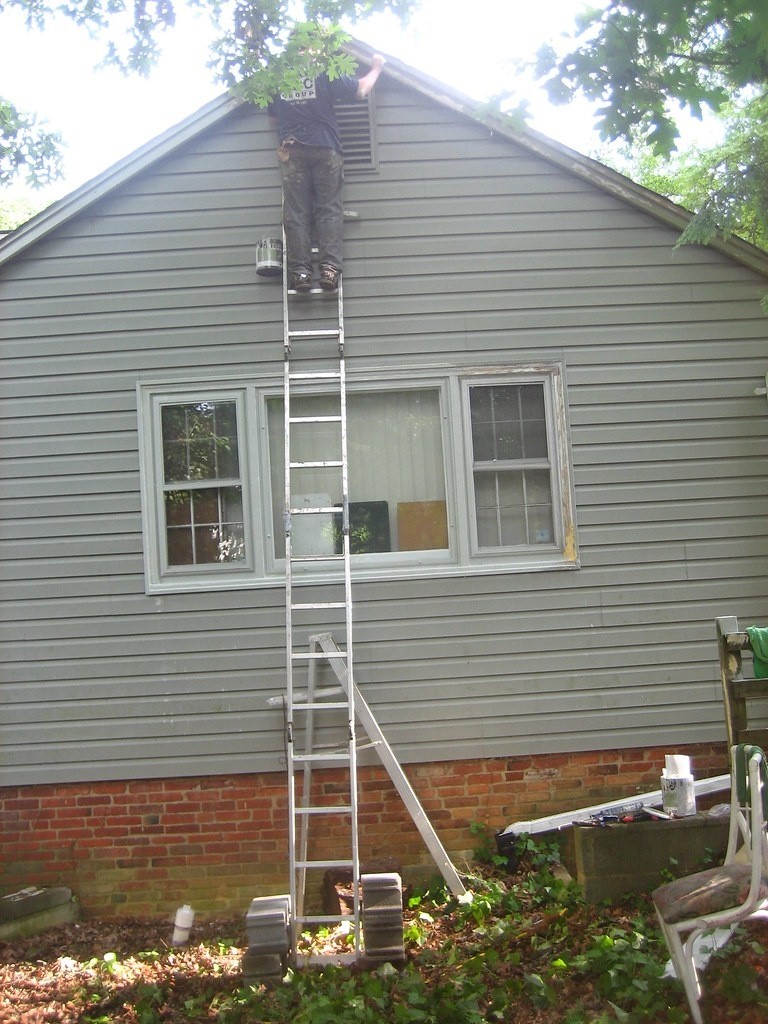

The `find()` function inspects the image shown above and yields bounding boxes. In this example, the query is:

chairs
[651,742,768,1024]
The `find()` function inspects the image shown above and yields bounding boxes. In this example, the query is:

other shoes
[287,271,313,291]
[318,269,340,289]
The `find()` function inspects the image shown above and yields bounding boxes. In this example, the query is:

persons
[265,29,384,291]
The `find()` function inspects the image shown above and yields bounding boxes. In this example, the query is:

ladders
[280,172,364,974]
